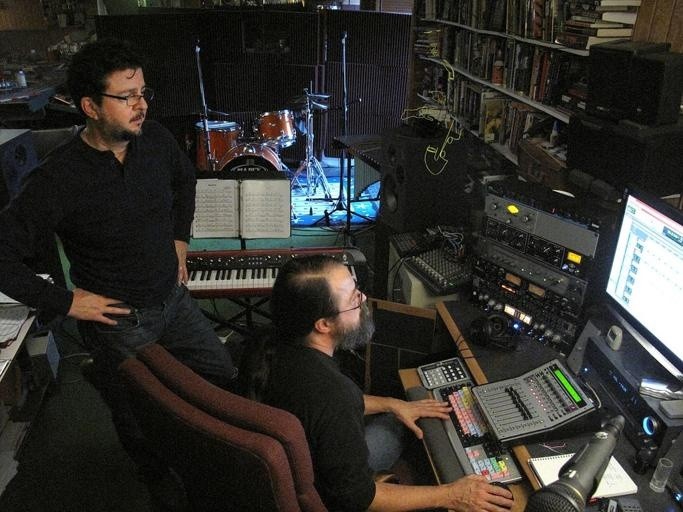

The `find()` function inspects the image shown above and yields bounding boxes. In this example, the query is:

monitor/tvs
[597,181,683,384]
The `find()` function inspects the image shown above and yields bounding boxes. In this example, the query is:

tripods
[291,113,333,202]
[310,45,376,226]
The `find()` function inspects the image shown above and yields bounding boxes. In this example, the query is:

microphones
[524,414,630,512]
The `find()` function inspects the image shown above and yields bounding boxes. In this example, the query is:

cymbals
[298,100,328,111]
[191,109,230,117]
[288,94,330,103]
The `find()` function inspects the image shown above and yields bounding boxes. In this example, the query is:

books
[191,178,293,239]
[526,451,639,502]
[414,1,642,155]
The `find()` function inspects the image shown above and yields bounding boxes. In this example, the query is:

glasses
[102,87,155,106]
[337,280,362,313]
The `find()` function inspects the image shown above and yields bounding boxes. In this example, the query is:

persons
[238,252,516,512]
[0,39,235,512]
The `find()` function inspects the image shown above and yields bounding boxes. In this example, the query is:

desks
[331,130,385,235]
[384,241,460,309]
[0,49,85,123]
[364,297,682,512]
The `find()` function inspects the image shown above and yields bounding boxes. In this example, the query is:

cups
[649,458,673,493]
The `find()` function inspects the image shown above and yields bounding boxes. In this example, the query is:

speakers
[585,41,667,126]
[626,51,683,126]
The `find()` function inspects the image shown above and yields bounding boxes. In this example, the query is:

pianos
[181,246,359,299]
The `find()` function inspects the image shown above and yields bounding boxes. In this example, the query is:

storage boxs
[516,137,568,192]
[24,328,60,383]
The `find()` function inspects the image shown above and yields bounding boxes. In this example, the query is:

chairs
[113,340,331,512]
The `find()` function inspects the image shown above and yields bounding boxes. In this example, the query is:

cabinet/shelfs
[0,281,51,498]
[403,0,590,170]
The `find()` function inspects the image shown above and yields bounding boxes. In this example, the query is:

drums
[218,143,283,176]
[252,108,296,148]
[193,119,241,174]
